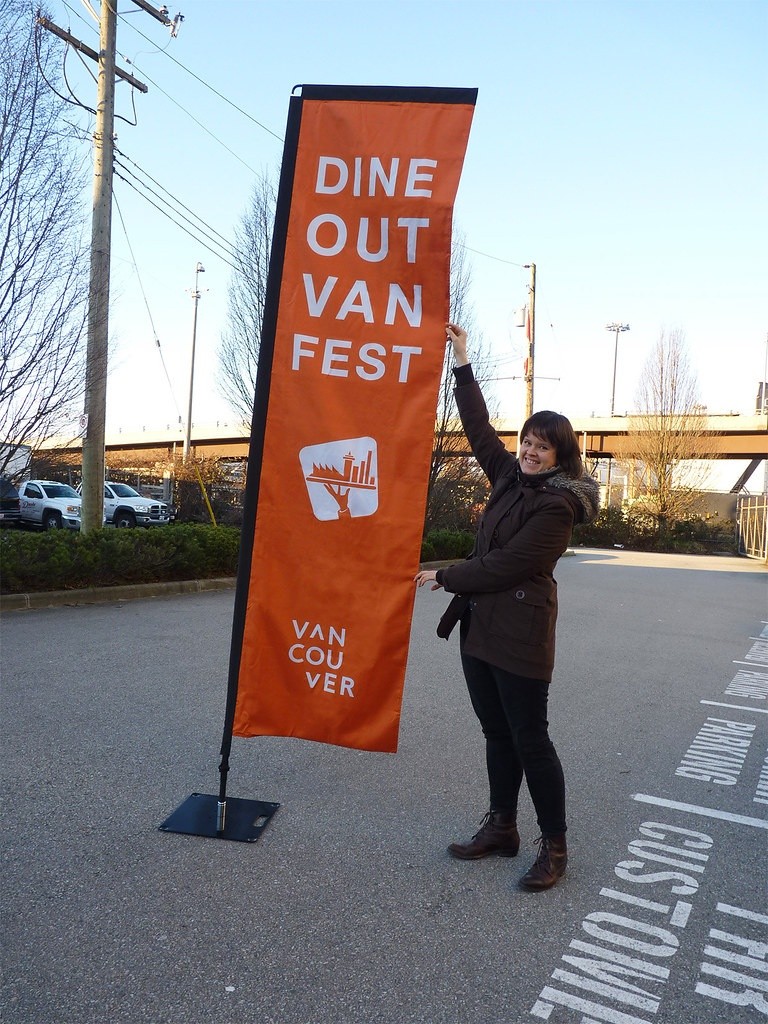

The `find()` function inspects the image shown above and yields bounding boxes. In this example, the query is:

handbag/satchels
[437,592,467,641]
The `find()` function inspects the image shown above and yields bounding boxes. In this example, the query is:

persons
[411,321,599,891]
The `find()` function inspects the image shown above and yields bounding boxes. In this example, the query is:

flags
[230,86,481,754]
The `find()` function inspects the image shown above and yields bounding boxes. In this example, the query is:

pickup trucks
[17,479,107,533]
[73,479,172,529]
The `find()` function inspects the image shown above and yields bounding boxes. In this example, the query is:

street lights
[180,260,209,466]
[606,323,631,418]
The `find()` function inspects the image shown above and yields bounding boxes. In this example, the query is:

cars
[0,474,23,520]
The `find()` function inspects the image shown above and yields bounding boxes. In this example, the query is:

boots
[518,832,568,891]
[447,810,520,860]
